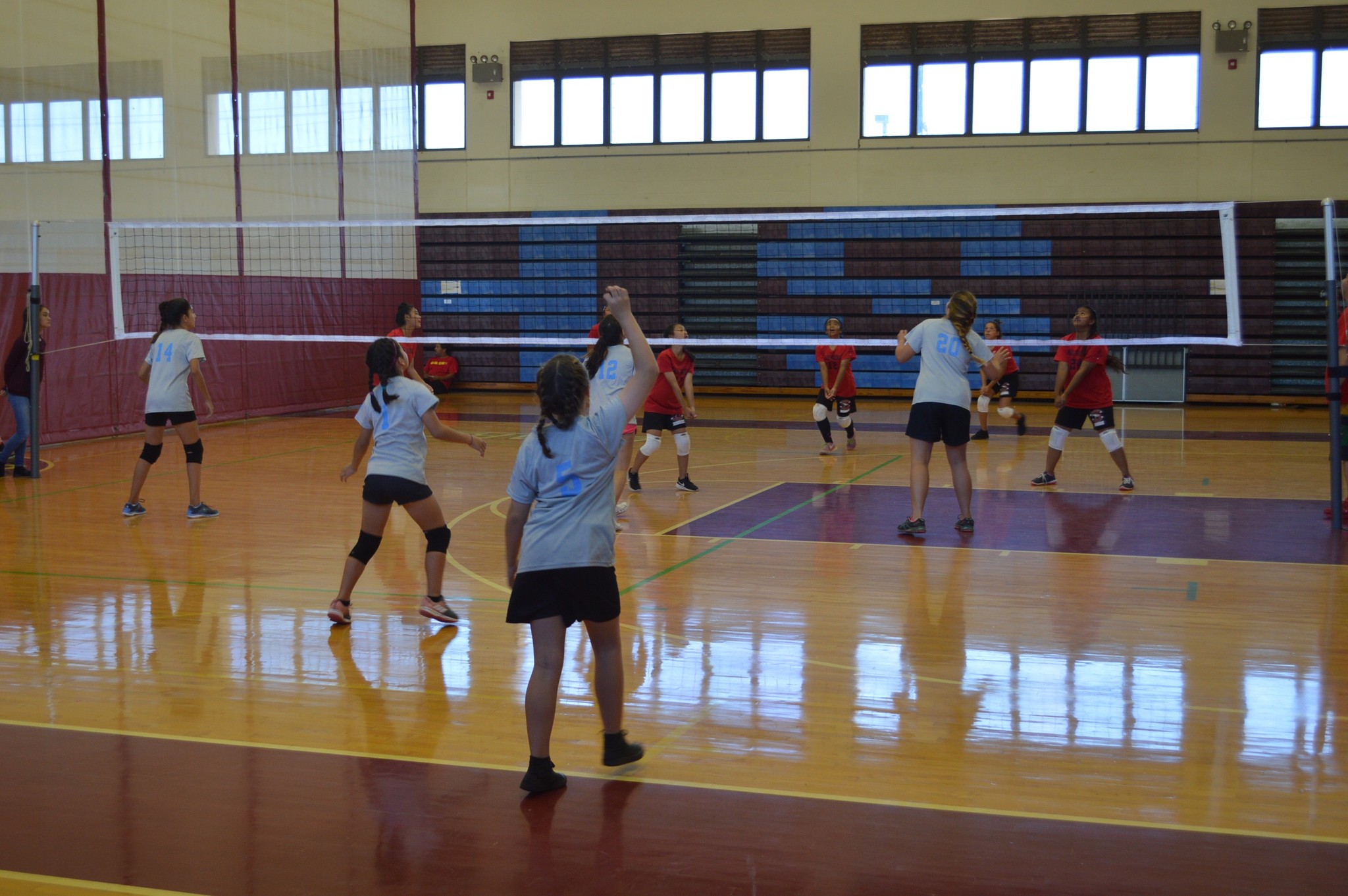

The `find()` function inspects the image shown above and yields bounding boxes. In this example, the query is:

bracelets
[468,433,474,445]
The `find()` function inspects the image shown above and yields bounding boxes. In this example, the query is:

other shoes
[0,461,5,476]
[13,467,41,477]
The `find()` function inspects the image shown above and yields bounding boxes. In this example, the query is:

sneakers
[1119,475,1135,490]
[327,599,352,624]
[122,501,147,516]
[675,476,699,491]
[846,427,856,450]
[614,520,623,531]
[971,429,989,439]
[1016,412,1026,435]
[603,731,643,767]
[954,517,974,531]
[418,596,459,623]
[819,442,837,454]
[519,755,567,792]
[614,501,628,514]
[897,514,926,533]
[1324,497,1348,519]
[1031,472,1057,485]
[186,502,220,517]
[627,468,641,491]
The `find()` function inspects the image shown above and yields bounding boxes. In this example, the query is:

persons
[420,343,459,393]
[627,321,699,492]
[812,317,858,456]
[504,284,661,794]
[1030,304,1135,490]
[1324,274,1348,519]
[327,338,488,625]
[970,318,1027,441]
[587,306,612,357]
[0,303,51,478]
[370,302,434,396]
[895,290,1010,534]
[581,314,638,530]
[121,298,221,518]
